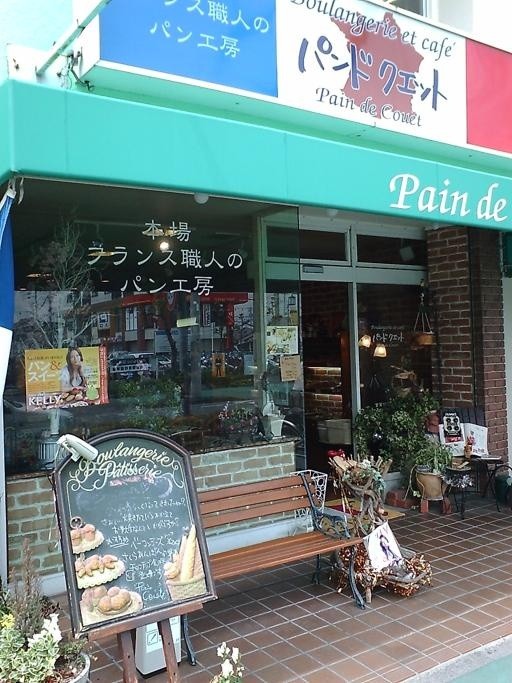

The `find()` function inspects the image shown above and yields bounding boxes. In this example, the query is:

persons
[379,534,398,562]
[58,346,88,393]
[216,356,221,376]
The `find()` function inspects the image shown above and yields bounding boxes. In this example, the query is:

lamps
[357,328,389,358]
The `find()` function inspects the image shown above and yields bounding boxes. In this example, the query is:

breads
[163,525,203,581]
[70,524,132,613]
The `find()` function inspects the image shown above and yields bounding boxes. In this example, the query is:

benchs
[181,472,380,666]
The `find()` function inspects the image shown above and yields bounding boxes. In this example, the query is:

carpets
[320,497,404,535]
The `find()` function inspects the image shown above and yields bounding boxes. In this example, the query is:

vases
[416,472,453,500]
[60,647,91,683]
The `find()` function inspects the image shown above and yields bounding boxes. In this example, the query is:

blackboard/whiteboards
[54,429,218,640]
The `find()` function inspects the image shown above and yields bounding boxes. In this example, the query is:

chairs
[438,406,504,519]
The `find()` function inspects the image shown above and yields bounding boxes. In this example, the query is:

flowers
[351,386,452,502]
[0,539,63,683]
